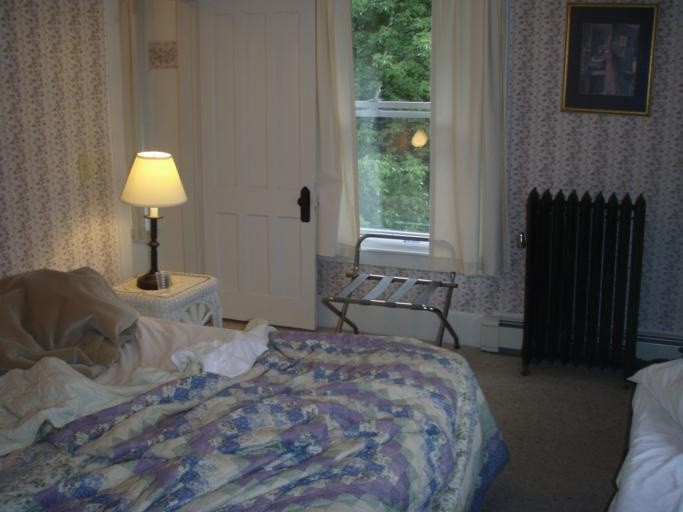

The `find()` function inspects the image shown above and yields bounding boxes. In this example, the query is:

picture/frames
[558,1,657,118]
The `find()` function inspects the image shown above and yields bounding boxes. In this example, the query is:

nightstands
[113,271,224,328]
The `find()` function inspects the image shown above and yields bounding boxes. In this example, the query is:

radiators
[516,187,648,388]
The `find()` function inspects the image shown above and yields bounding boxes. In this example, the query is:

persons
[599,48,618,97]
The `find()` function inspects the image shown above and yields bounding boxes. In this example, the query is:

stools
[316,232,460,350]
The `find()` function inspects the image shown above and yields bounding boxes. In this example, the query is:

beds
[0,324,472,510]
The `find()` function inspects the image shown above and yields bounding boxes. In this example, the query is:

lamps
[119,149,188,290]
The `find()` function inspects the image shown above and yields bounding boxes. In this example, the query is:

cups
[155,270,173,294]
